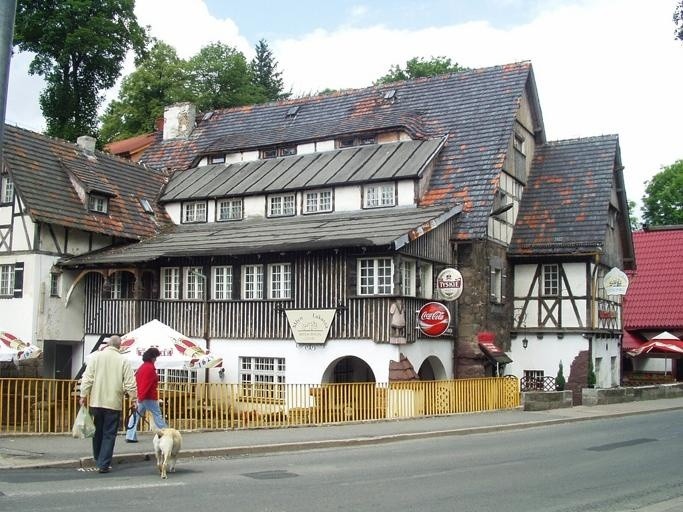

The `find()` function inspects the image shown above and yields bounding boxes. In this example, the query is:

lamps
[522,322,528,348]
[490,203,514,216]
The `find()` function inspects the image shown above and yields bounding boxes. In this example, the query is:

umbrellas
[81,318,223,370]
[0,331,42,362]
[626,330,682,377]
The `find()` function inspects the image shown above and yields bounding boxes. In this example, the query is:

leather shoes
[124,439,138,443]
[100,466,113,473]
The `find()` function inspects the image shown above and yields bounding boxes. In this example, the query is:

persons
[125,349,170,444]
[78,334,139,473]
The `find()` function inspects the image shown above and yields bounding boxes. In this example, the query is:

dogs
[147,409,182,480]
